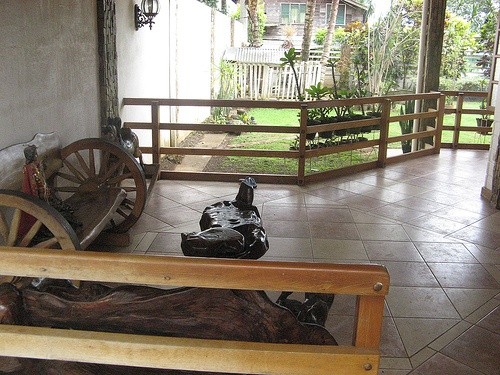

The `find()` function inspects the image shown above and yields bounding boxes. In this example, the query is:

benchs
[26,187,127,250]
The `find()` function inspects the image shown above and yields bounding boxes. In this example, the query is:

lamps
[134,0,160,31]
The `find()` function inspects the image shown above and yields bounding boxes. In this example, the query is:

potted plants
[399,99,415,153]
[476,79,495,135]
[280,48,399,140]
